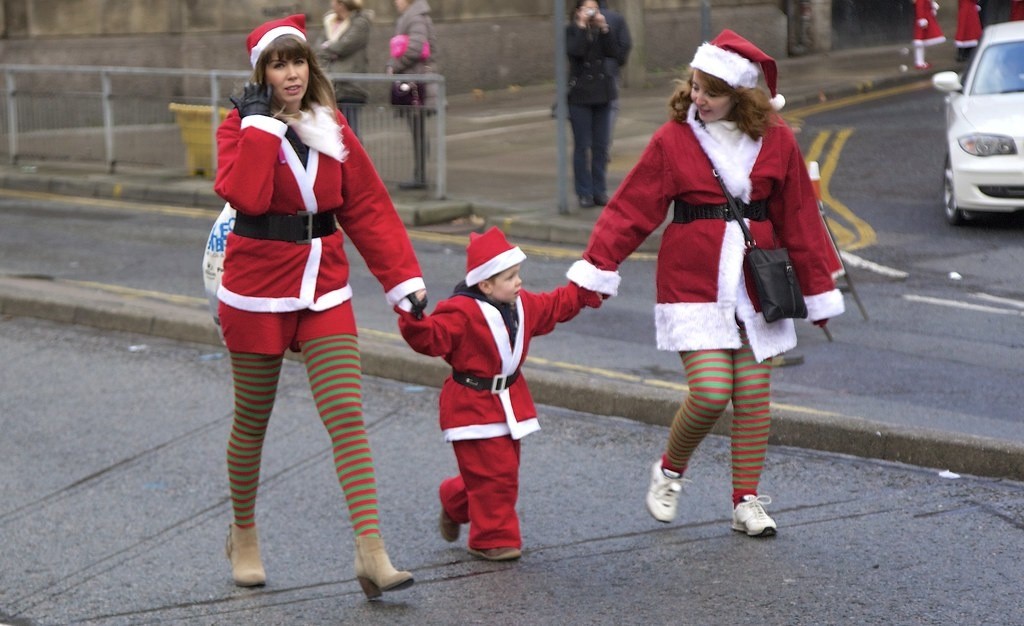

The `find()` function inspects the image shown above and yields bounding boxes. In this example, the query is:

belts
[672,196,770,225]
[452,368,521,394]
[234,208,336,243]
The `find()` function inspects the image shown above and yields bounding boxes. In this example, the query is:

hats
[690,28,786,111]
[246,12,306,68]
[465,227,527,288]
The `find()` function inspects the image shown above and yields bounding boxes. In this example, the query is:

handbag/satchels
[391,81,422,116]
[746,246,808,322]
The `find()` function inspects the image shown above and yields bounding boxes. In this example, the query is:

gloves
[229,81,274,117]
[406,293,428,319]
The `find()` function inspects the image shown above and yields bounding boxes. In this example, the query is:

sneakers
[733,494,777,536]
[646,458,688,523]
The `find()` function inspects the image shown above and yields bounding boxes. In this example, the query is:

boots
[351,536,414,599]
[226,524,266,586]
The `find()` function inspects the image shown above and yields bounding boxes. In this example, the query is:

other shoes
[466,544,521,560]
[917,64,931,70]
[597,193,611,205]
[580,195,594,207]
[439,506,460,542]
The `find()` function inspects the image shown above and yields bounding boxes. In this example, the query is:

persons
[567,0,632,207]
[314,0,374,141]
[395,226,601,559]
[953,0,982,62]
[213,15,425,599]
[911,0,946,70]
[563,28,844,536]
[385,0,450,190]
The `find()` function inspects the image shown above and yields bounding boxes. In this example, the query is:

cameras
[585,9,595,16]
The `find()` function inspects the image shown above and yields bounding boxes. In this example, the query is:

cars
[931,20,1024,228]
